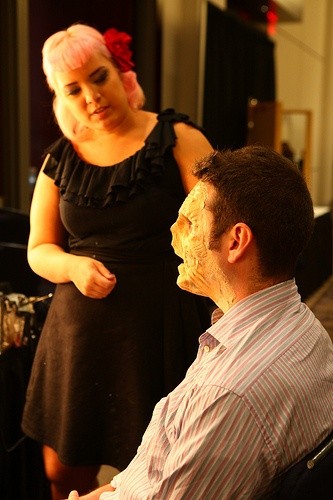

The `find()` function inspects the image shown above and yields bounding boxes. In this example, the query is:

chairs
[259,426,332,500]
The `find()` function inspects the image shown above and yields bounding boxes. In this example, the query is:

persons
[62,146,330,498]
[22,23,219,499]
